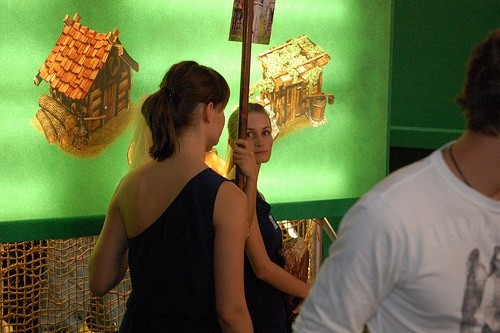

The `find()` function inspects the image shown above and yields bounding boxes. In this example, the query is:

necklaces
[447,144,500,199]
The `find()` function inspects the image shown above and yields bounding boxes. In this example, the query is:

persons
[86,61,255,333]
[225,102,310,333]
[291,30,500,332]
